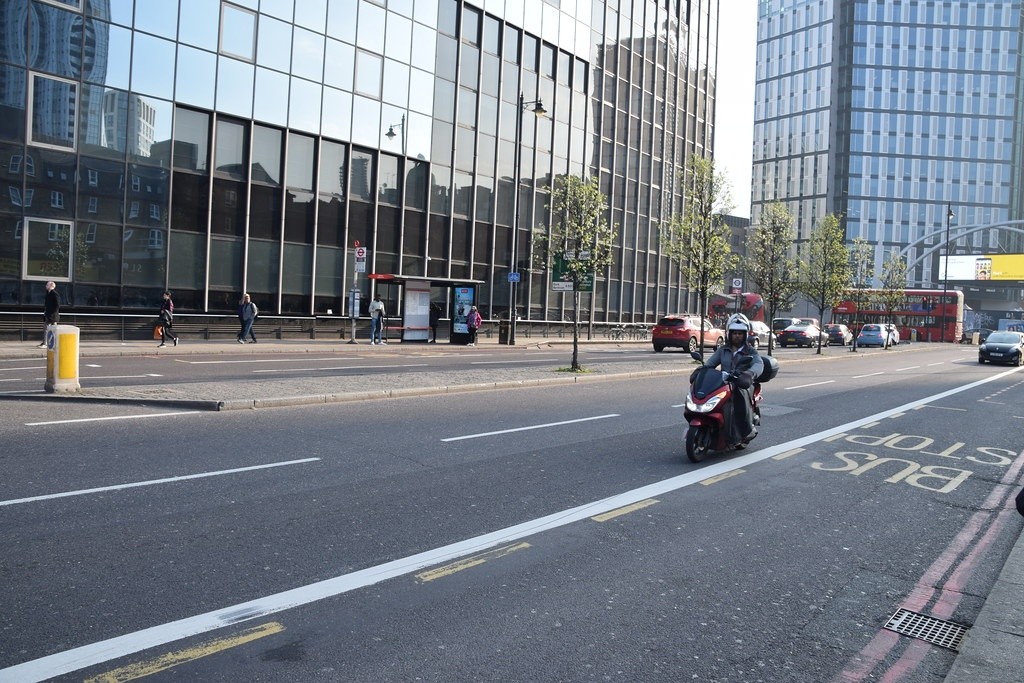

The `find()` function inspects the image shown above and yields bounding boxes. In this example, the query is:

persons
[430,305,439,343]
[369,294,385,345]
[239,294,258,344]
[87,291,98,306]
[690,314,763,440]
[467,306,481,345]
[38,281,60,348]
[158,292,178,347]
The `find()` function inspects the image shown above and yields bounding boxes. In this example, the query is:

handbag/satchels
[153,326,165,339]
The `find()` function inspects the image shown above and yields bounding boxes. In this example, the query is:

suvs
[651,313,725,354]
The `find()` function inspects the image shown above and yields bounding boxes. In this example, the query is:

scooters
[683,352,781,464]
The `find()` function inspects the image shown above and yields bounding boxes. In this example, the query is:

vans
[771,317,819,342]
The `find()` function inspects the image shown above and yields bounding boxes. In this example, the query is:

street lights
[700,230,724,357]
[385,112,405,154]
[508,91,548,345]
[940,201,957,342]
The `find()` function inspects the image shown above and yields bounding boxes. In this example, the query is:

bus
[709,292,765,323]
[831,288,964,343]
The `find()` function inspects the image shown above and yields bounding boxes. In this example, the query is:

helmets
[726,313,750,341]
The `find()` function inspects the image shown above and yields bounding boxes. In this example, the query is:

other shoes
[371,341,374,345]
[238,339,244,344]
[157,343,166,348]
[467,343,474,346]
[378,342,385,345]
[249,339,255,343]
[37,343,48,348]
[174,337,178,346]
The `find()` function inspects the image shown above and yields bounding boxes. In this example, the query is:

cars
[821,324,853,347]
[883,323,900,345]
[857,323,892,347]
[979,330,1024,366]
[778,324,831,348]
[747,321,778,350]
